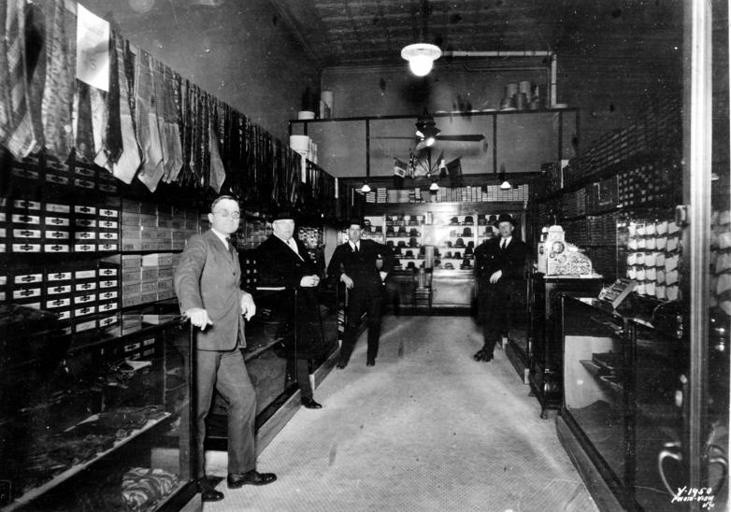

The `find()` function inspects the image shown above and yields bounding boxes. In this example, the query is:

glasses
[214,209,241,221]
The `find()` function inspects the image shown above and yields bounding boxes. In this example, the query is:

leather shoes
[196,477,224,502]
[337,361,348,369]
[301,397,322,409]
[228,471,276,488]
[366,358,375,367]
[474,347,494,362]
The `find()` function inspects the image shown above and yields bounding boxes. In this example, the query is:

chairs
[411,264,436,313]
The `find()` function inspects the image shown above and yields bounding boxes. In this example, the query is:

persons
[327,218,394,368]
[472,212,526,363]
[174,194,276,501]
[260,206,320,408]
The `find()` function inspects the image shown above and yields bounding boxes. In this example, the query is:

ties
[355,245,358,253]
[501,239,507,249]
[225,237,234,258]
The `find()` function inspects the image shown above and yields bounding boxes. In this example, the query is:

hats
[406,262,415,268]
[386,216,419,234]
[349,218,364,225]
[478,215,519,233]
[386,238,418,256]
[394,259,401,266]
[267,208,295,223]
[444,217,474,269]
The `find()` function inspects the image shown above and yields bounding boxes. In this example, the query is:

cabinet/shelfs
[0,286,303,512]
[508,266,727,511]
[382,202,528,311]
